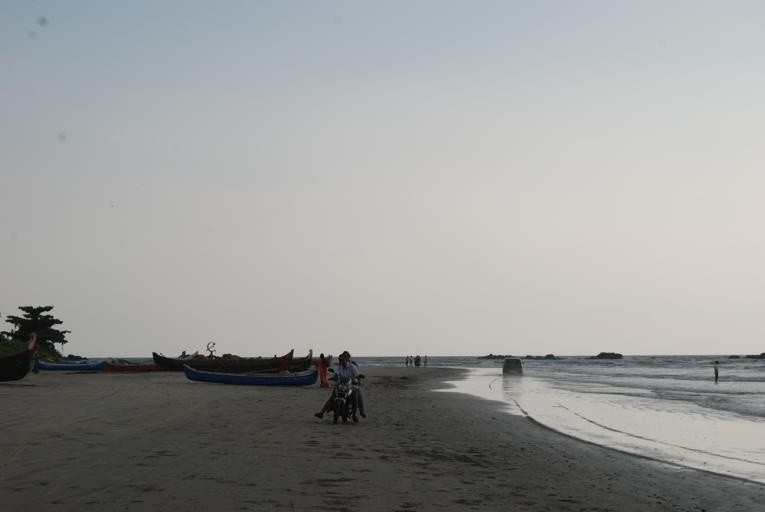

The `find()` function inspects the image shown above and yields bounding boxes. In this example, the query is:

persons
[405,354,421,368]
[315,353,365,422]
[316,353,331,388]
[423,354,428,367]
[341,351,368,417]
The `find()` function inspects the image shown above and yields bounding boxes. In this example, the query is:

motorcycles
[327,368,365,425]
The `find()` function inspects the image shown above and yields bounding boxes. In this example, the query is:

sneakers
[316,412,323,418]
[352,413,366,422]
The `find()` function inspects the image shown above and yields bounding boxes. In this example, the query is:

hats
[338,355,345,360]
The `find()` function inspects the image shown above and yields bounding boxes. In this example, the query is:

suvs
[500,357,525,375]
